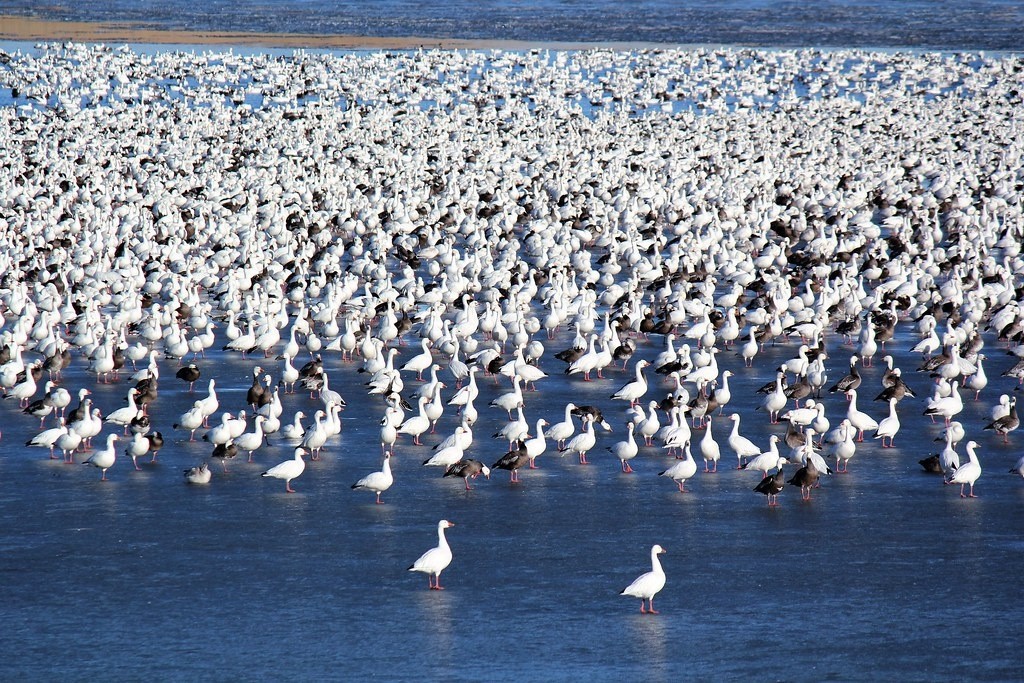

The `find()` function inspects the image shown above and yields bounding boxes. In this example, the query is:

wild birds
[1,41,1023,507]
[406,519,456,590]
[619,544,666,614]
